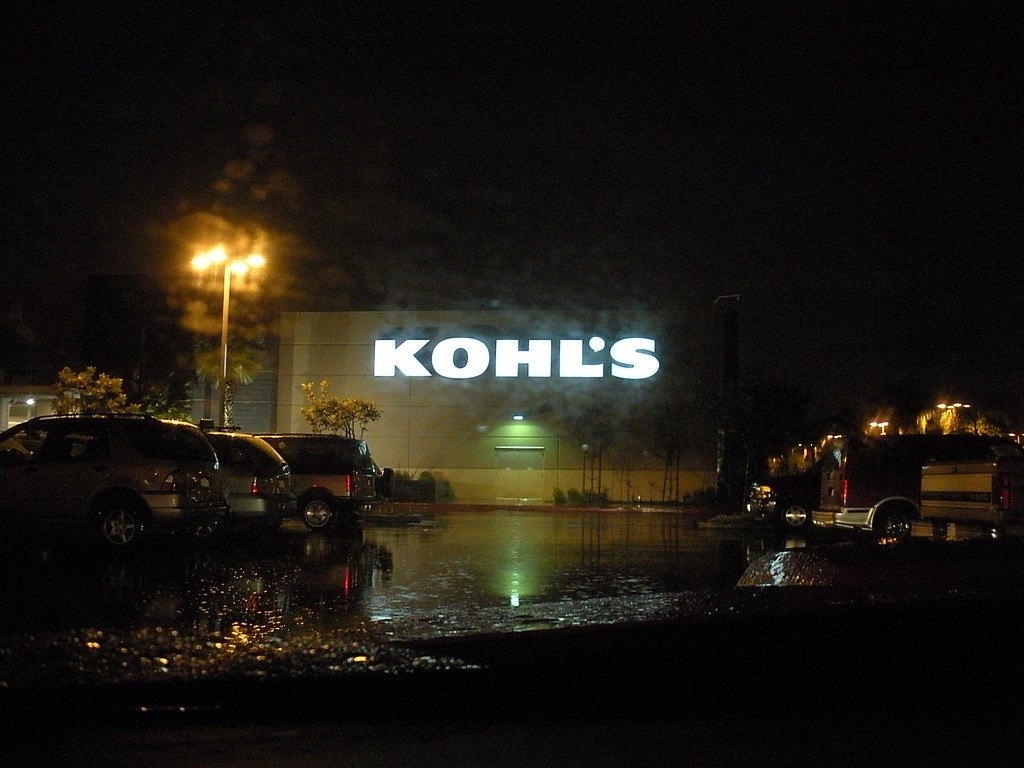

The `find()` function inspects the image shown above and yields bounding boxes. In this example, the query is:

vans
[745,431,1024,555]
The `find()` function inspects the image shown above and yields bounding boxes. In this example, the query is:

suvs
[246,432,383,531]
[200,418,291,535]
[0,412,229,557]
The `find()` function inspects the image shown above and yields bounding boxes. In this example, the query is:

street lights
[191,251,268,432]
